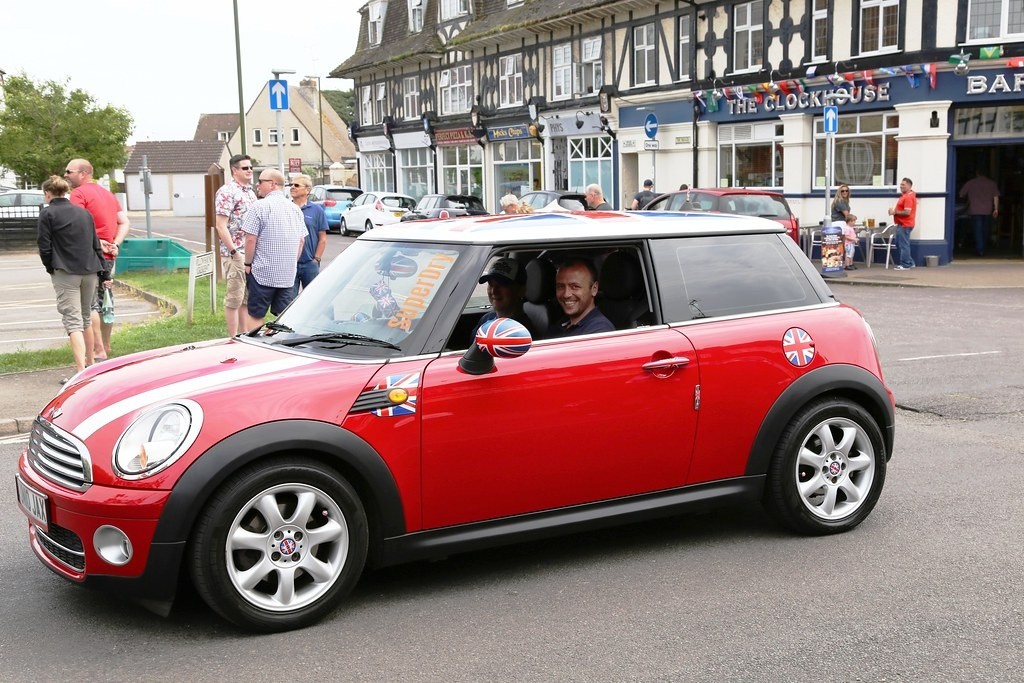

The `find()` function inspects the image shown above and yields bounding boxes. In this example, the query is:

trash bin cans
[821,227,847,277]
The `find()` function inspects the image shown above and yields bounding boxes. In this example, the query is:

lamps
[536,114,553,133]
[712,77,734,100]
[767,69,791,94]
[832,60,857,86]
[930,111,939,128]
[575,110,593,129]
[954,47,969,76]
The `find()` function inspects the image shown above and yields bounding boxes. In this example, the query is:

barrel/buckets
[925,256,939,266]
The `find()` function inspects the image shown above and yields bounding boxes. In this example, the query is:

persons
[290,173,329,299]
[543,257,615,342]
[500,194,518,214]
[64,158,131,361]
[241,168,310,331]
[533,178,540,190]
[679,184,690,210]
[214,154,258,337]
[842,214,862,270]
[955,165,1000,257]
[830,184,850,240]
[469,258,539,344]
[37,175,107,385]
[630,179,661,211]
[584,184,612,210]
[888,177,916,271]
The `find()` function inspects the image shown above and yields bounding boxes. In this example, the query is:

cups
[889,207,894,215]
[863,221,866,226]
[857,227,860,233]
[868,219,875,228]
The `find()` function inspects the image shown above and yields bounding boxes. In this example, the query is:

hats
[644,180,653,186]
[478,256,527,290]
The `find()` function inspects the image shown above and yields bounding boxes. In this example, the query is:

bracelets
[243,262,251,266]
[113,243,119,248]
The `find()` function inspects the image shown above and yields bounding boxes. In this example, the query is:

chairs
[596,249,649,329]
[523,256,563,339]
[807,224,898,269]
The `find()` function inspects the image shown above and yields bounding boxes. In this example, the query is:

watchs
[231,249,236,254]
[313,256,321,262]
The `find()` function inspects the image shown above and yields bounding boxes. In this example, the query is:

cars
[498,190,608,215]
[641,186,800,246]
[306,184,364,234]
[399,194,490,222]
[0,188,70,217]
[340,191,417,236]
[13,211,897,635]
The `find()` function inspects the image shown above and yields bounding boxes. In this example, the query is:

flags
[696,46,1024,112]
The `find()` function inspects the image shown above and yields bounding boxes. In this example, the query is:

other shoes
[845,266,854,270]
[849,263,857,270]
[976,252,983,257]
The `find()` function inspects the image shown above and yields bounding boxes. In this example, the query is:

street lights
[636,106,657,195]
[304,75,325,185]
[824,93,852,228]
[271,67,297,176]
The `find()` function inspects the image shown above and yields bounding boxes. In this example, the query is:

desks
[852,226,897,267]
[798,224,820,256]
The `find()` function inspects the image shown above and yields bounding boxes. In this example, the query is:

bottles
[102,288,115,323]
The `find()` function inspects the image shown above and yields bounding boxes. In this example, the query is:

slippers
[894,265,910,270]
[910,264,915,267]
[85,357,107,362]
[59,377,72,385]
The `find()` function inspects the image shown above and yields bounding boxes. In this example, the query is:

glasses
[841,190,848,192]
[290,184,306,188]
[66,169,83,174]
[235,167,252,170]
[258,179,273,184]
